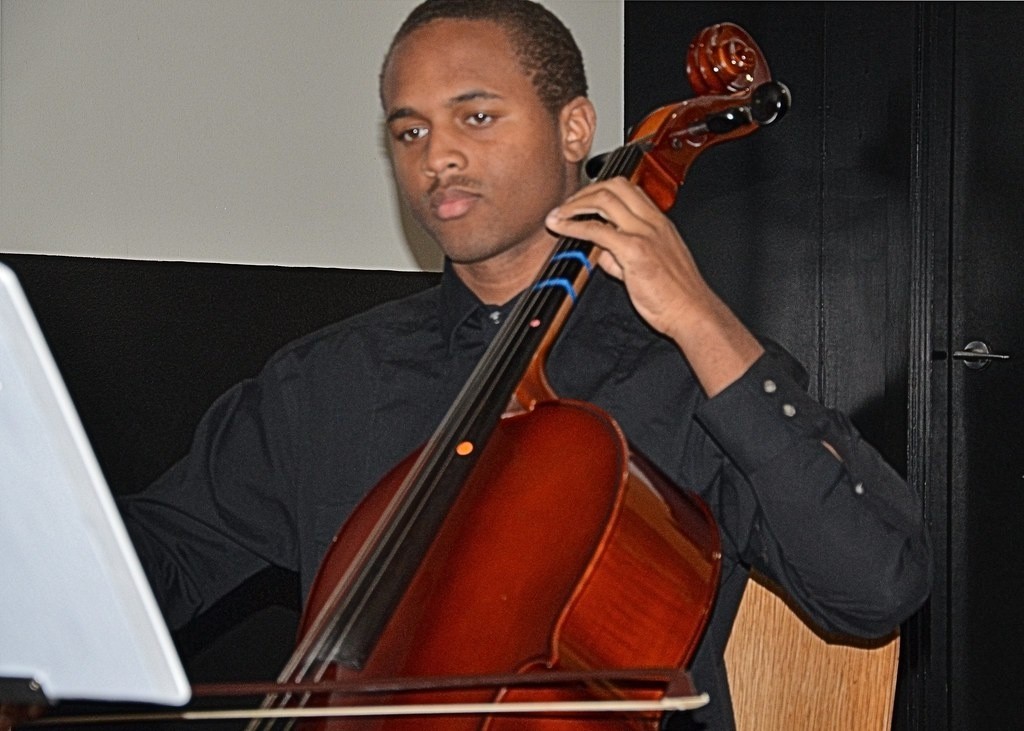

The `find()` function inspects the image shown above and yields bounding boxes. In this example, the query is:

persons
[114,0,937,731]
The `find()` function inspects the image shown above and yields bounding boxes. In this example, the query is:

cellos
[244,22,792,731]
